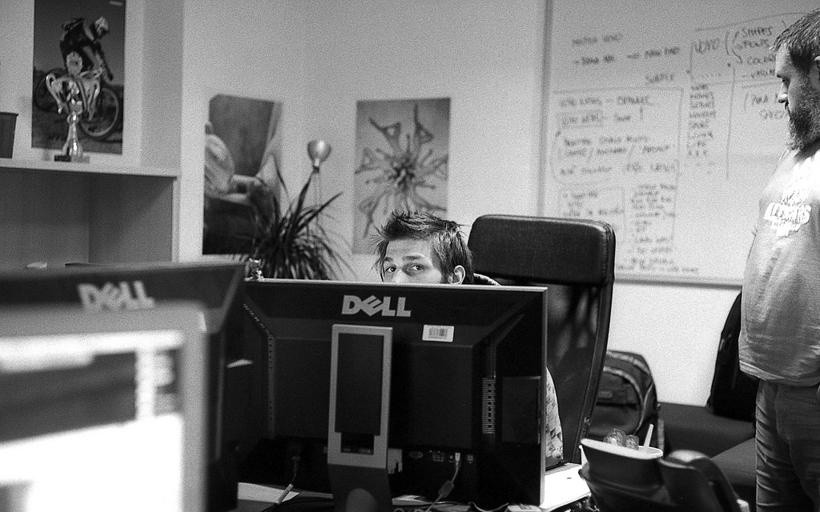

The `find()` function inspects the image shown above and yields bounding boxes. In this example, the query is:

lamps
[305,137,331,254]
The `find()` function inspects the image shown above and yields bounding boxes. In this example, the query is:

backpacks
[62,16,82,30]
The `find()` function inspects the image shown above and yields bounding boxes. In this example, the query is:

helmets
[95,17,108,39]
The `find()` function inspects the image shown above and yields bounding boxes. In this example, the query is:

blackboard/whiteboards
[536,0,820,288]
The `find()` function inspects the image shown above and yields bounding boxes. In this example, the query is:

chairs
[468,213,616,461]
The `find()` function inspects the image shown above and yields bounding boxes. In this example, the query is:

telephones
[657,449,743,512]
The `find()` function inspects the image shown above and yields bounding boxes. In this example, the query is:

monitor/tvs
[231,277,548,512]
[0,258,244,512]
[0,302,208,512]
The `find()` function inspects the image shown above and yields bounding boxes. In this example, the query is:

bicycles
[34,63,123,144]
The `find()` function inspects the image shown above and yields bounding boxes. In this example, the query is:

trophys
[45,51,100,164]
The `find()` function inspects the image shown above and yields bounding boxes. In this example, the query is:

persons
[60,16,113,81]
[739,7,820,512]
[374,209,564,470]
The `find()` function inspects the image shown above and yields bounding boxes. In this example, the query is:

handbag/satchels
[708,294,760,421]
[591,349,660,447]
